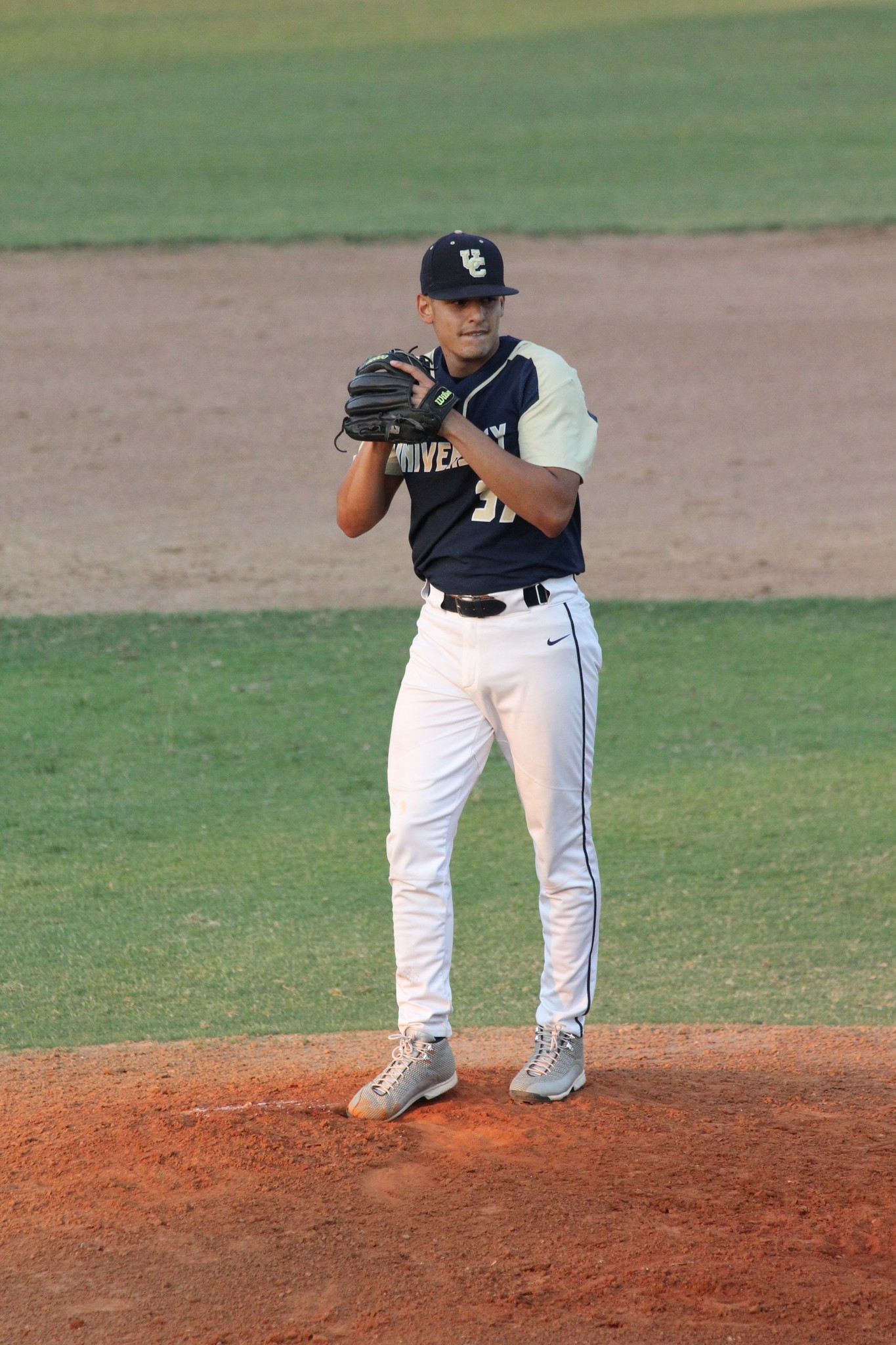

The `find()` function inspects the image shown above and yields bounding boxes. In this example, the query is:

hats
[419,226,519,299]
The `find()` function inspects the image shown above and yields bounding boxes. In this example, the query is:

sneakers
[508,1032,586,1104]
[346,1026,458,1123]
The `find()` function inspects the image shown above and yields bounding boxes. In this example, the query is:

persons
[330,231,604,1120]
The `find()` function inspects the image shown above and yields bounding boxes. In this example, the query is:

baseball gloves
[344,350,460,445]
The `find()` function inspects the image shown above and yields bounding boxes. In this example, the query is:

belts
[441,581,551,617]
